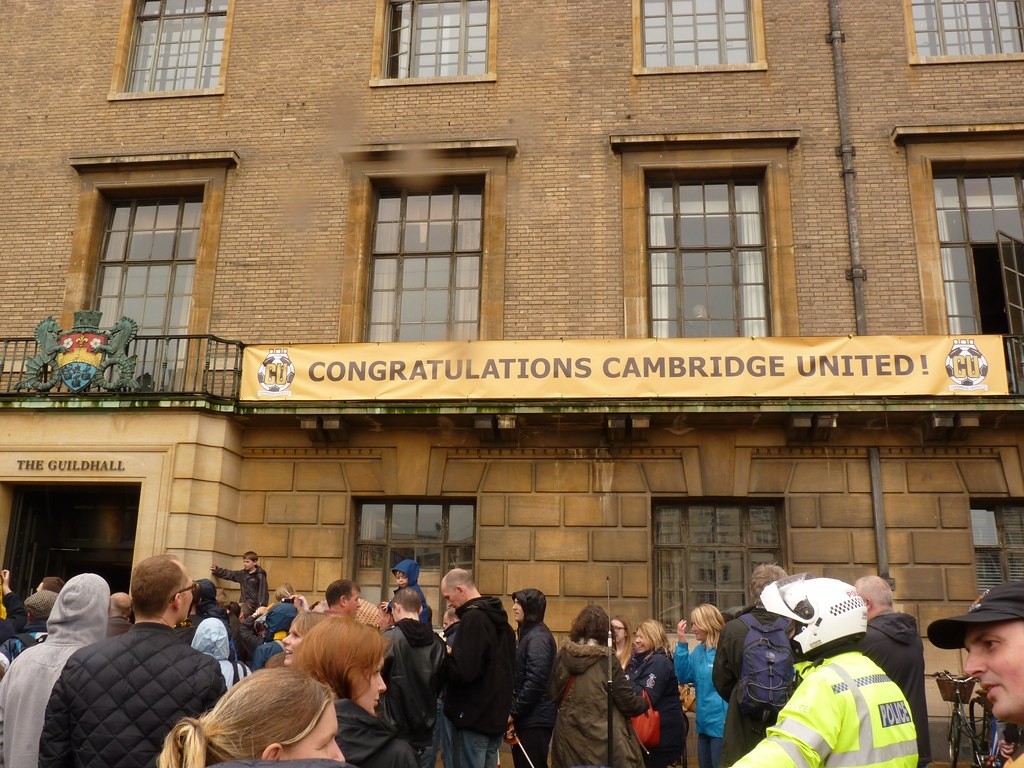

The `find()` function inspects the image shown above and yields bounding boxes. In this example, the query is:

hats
[926,580,1024,650]
[24,589,59,620]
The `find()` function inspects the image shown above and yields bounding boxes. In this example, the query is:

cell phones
[282,598,295,604]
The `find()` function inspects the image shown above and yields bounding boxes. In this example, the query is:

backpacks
[736,612,795,723]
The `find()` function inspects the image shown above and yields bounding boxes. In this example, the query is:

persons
[0,552,1024,768]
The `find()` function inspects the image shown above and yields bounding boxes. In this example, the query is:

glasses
[168,582,195,603]
[614,626,626,633]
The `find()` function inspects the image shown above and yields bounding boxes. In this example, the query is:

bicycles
[931,670,994,768]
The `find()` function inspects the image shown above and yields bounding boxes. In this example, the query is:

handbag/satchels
[629,689,660,748]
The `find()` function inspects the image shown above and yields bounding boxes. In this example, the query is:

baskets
[936,673,976,704]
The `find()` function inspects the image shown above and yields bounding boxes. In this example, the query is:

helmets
[760,572,868,656]
